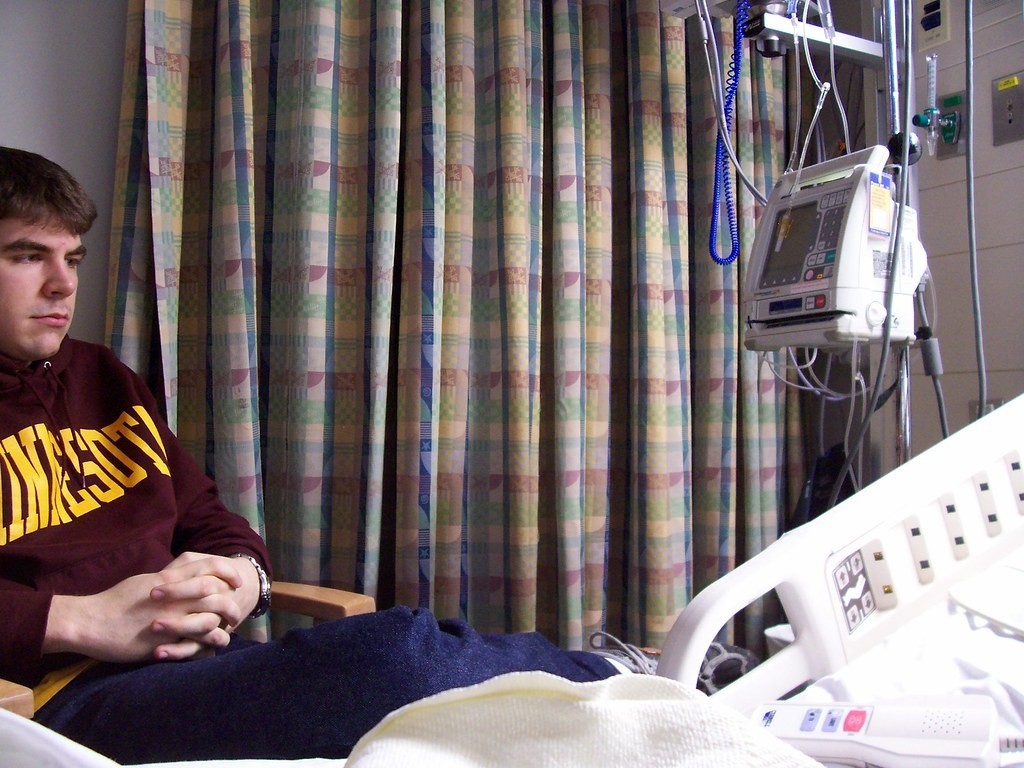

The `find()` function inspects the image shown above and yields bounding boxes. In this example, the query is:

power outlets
[990,70,1024,147]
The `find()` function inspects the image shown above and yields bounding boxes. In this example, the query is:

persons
[0,143,764,765]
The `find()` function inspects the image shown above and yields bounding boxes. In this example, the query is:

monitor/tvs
[742,143,927,353]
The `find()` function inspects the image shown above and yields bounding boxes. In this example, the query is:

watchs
[232,551,273,622]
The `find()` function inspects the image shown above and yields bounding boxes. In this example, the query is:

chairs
[2,576,378,719]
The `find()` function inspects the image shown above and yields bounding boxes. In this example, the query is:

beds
[1,392,1024,768]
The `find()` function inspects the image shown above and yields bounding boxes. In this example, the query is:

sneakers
[587,632,759,696]
